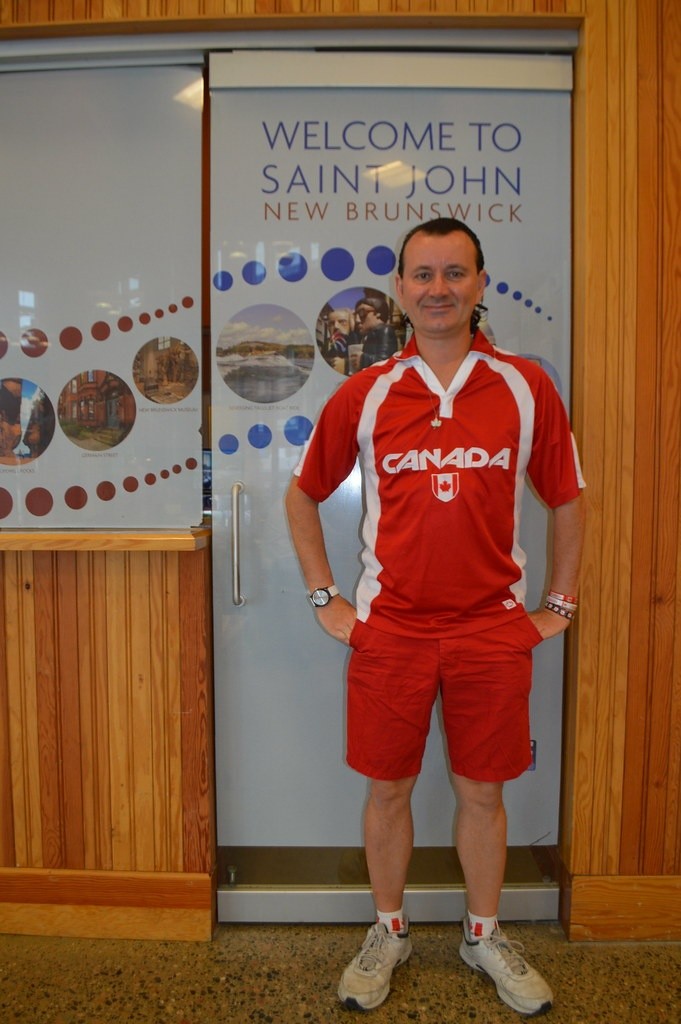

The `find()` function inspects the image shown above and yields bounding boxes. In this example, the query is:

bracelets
[544,590,578,620]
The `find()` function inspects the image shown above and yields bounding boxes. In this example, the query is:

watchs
[307,584,340,607]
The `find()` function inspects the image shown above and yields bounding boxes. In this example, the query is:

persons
[322,298,398,376]
[285,217,587,1016]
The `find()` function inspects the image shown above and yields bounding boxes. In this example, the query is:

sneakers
[337,913,413,1011]
[459,916,554,1017]
